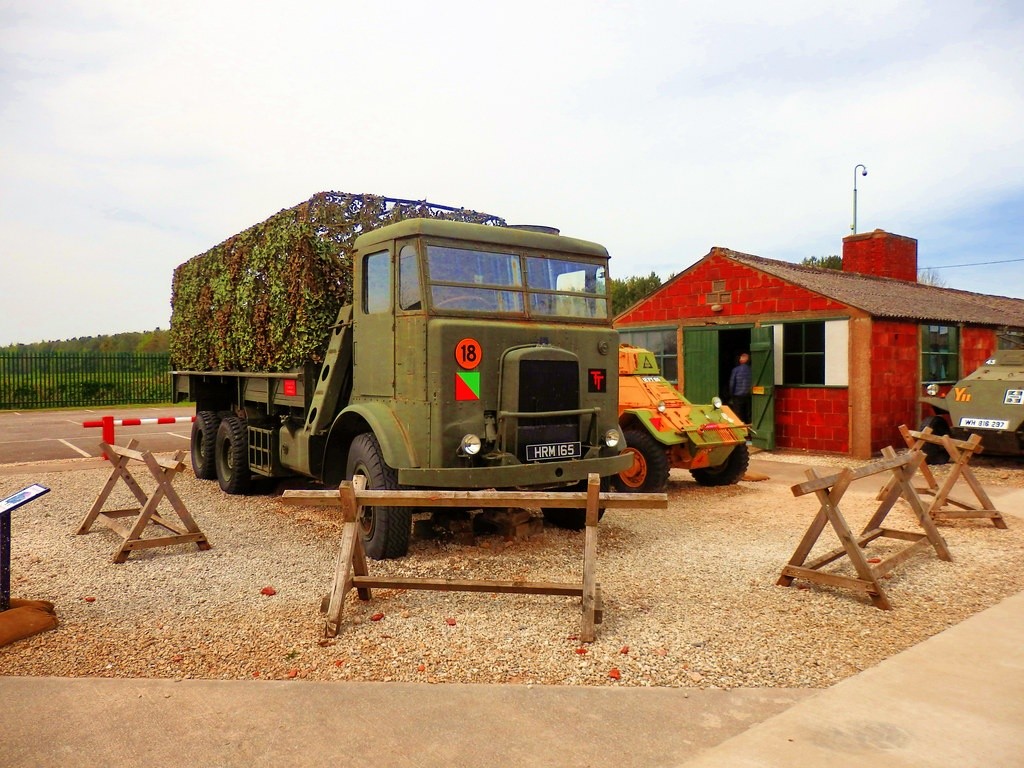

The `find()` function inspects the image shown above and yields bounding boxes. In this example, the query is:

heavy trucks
[167,192,633,562]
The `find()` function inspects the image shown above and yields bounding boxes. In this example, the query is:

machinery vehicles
[608,343,750,493]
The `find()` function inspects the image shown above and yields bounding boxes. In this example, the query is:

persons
[727,353,752,441]
